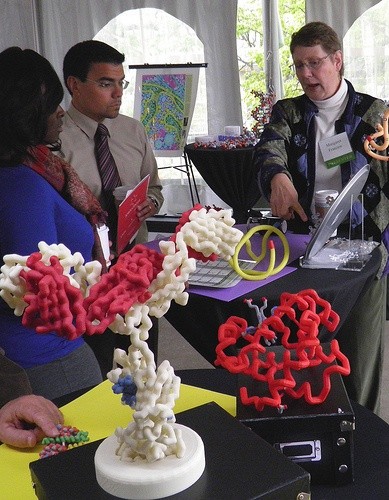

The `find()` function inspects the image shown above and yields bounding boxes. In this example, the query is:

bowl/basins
[194,135,216,144]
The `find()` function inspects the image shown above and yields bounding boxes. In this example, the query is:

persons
[0,47,113,400]
[252,21,389,418]
[46,40,163,366]
[0,349,67,448]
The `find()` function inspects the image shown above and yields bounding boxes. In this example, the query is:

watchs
[149,196,159,207]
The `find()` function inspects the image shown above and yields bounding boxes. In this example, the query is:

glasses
[288,49,334,77]
[86,79,130,90]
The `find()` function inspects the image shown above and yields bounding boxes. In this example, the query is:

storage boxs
[235,362,355,488]
[26,401,312,500]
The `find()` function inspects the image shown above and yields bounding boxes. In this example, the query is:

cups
[224,125,241,137]
[315,190,339,237]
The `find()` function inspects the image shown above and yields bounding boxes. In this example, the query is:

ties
[94,124,124,206]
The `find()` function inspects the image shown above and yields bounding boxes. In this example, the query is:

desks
[29,370,389,500]
[184,143,262,225]
[163,220,387,418]
